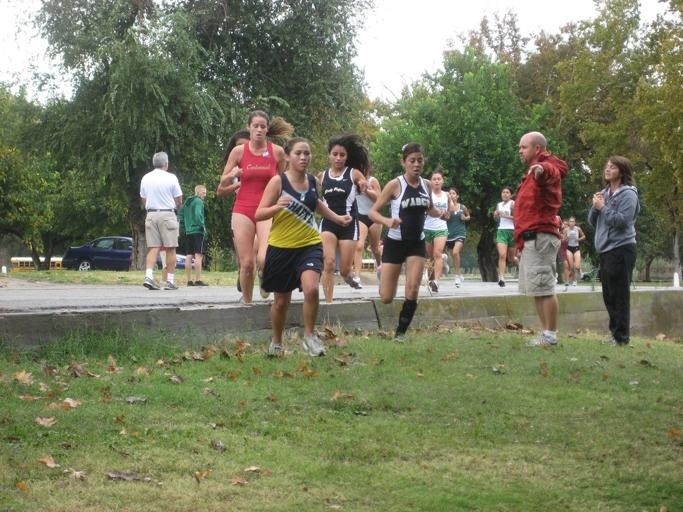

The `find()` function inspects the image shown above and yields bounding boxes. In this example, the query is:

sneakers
[353,278,362,289]
[497,280,505,287]
[442,253,449,273]
[143,276,161,290]
[454,274,464,288]
[259,273,270,298]
[532,334,557,345]
[164,281,179,290]
[269,341,285,355]
[302,333,326,357]
[599,338,625,347]
[429,279,439,292]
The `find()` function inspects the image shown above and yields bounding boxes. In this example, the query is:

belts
[148,209,172,212]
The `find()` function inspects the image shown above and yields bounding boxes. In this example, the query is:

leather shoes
[194,281,209,286]
[188,281,195,286]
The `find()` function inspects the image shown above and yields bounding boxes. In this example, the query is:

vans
[351,259,376,272]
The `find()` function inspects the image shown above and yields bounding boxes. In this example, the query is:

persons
[184,185,208,286]
[587,155,640,346]
[494,187,585,286]
[513,131,568,345]
[139,151,183,290]
[216,107,471,357]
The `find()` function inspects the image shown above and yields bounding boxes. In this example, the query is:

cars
[579,269,600,281]
[62,235,196,271]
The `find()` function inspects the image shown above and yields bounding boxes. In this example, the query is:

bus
[9,257,62,273]
